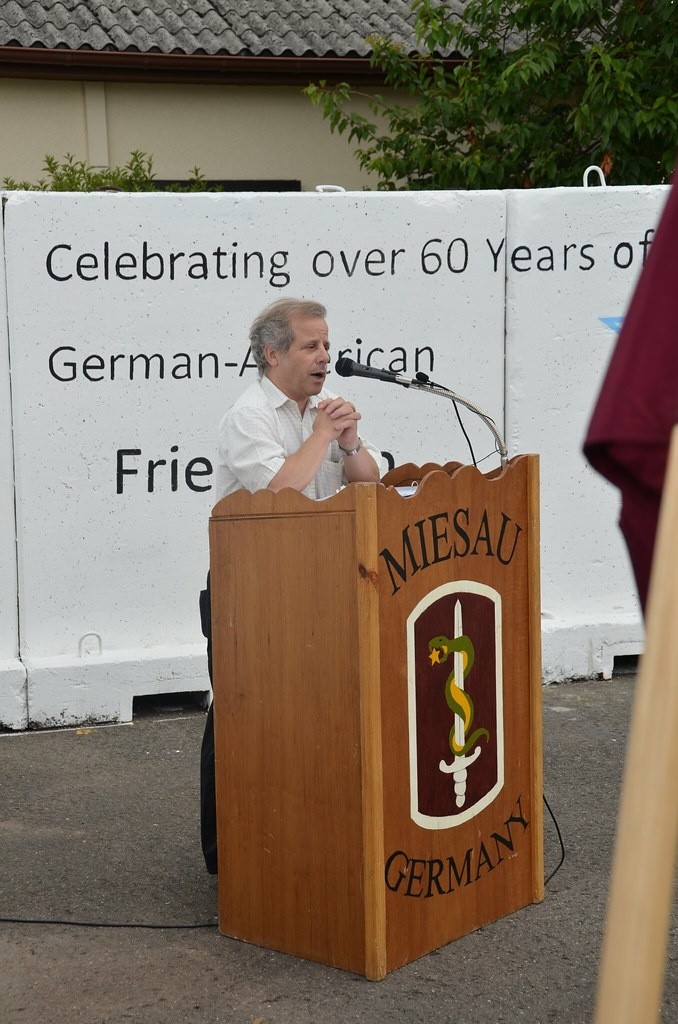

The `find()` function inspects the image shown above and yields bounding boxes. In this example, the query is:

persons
[198,297,381,873]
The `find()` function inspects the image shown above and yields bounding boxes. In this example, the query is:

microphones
[416,372,429,383]
[335,358,420,385]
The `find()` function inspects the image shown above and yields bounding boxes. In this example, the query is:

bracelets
[339,437,362,456]
